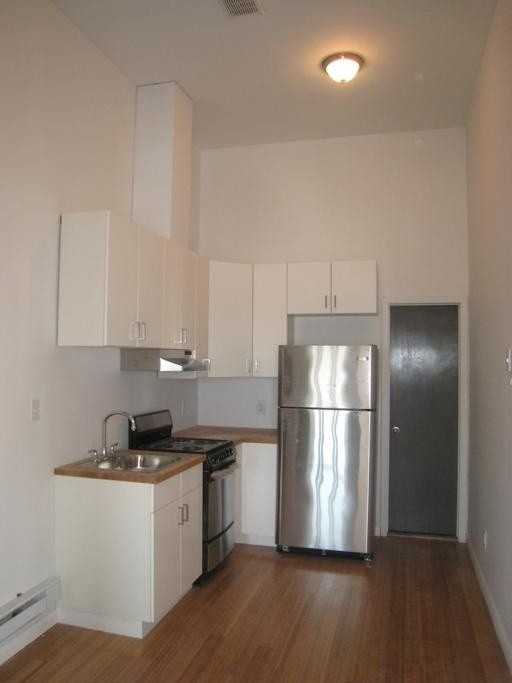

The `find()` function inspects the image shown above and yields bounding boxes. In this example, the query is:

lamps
[322,53,363,82]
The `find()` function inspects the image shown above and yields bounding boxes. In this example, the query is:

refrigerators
[275,345,379,561]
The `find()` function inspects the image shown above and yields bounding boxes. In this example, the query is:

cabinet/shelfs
[164,238,195,352]
[195,253,208,367]
[57,210,164,350]
[240,441,279,547]
[208,261,288,380]
[54,460,204,624]
[288,260,379,315]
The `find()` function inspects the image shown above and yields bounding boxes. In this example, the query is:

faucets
[100,411,136,456]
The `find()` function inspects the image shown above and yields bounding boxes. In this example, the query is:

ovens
[203,465,235,573]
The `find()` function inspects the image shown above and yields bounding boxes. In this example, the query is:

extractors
[160,351,208,372]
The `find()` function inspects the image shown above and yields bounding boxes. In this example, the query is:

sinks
[77,447,184,473]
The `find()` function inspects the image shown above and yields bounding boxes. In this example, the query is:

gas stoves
[150,436,233,454]
